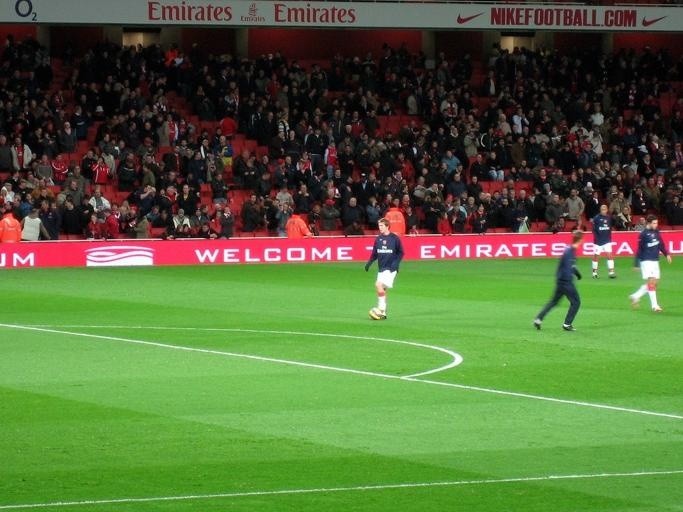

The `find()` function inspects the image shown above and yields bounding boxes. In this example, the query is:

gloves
[576,271,582,281]
[364,264,370,271]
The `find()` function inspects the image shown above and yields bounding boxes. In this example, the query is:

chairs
[0,32,683,240]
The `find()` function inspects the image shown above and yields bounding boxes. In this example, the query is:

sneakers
[372,308,386,319]
[629,294,663,312]
[563,323,576,331]
[533,322,541,331]
[592,273,618,279]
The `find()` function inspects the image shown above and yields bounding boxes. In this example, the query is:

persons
[533,230,581,332]
[0,37,683,242]
[363,217,404,321]
[591,205,616,280]
[628,217,670,312]
